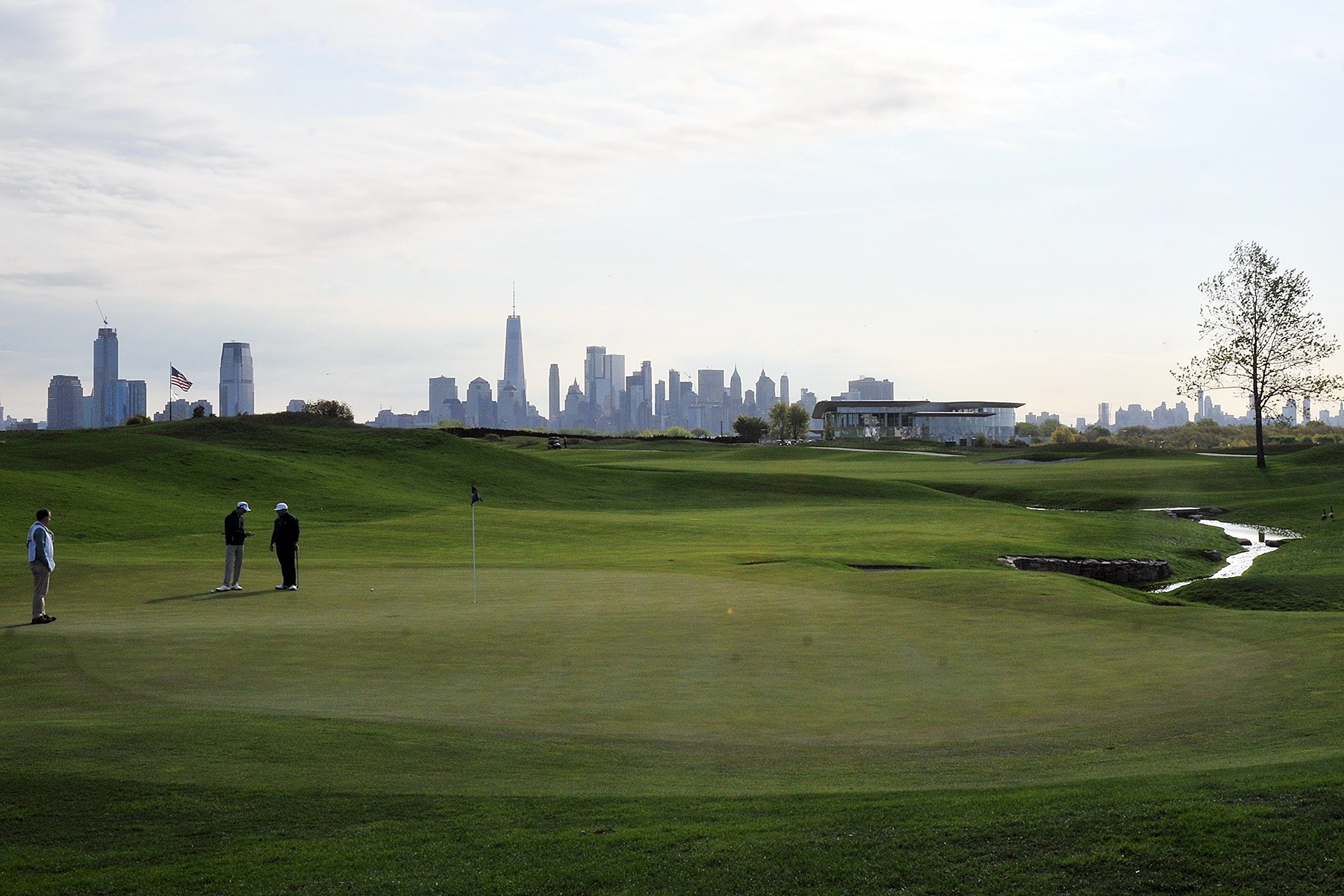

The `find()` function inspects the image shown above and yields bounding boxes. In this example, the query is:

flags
[171,365,193,392]
[471,485,480,503]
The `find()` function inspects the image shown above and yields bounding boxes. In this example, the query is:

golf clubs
[221,533,255,535]
[296,551,299,588]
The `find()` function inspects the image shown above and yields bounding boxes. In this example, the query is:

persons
[26,509,57,624]
[269,503,300,591]
[563,435,567,448]
[216,502,252,591]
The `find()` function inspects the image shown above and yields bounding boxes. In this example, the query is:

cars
[776,440,791,446]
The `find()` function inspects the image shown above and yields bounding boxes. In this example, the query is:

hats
[273,503,288,510]
[237,502,251,511]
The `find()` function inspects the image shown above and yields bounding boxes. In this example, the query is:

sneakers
[276,584,288,590]
[289,585,297,591]
[231,584,242,591]
[216,585,230,592]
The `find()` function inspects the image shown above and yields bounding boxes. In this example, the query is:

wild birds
[1321,506,1335,522]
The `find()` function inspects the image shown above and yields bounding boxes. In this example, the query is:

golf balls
[371,588,374,591]
[320,508,323,510]
[211,590,214,593]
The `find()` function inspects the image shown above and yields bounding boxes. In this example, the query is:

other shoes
[31,614,57,624]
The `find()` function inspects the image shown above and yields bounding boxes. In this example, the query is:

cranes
[96,300,108,427]
[683,371,691,382]
